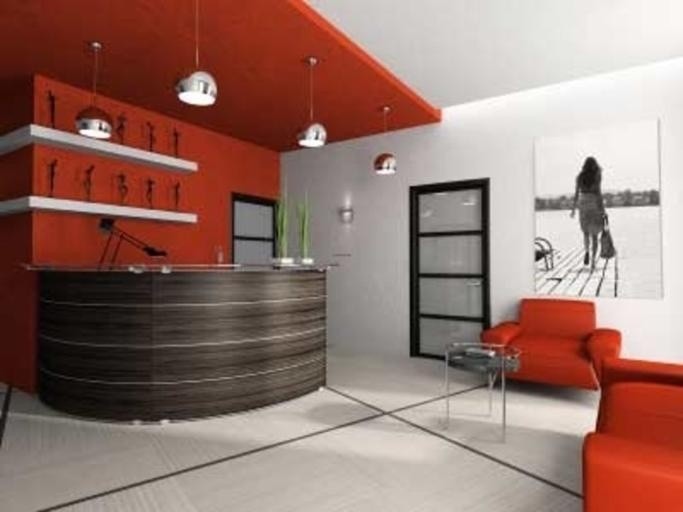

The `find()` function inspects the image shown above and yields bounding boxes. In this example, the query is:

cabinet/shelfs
[0,124,199,227]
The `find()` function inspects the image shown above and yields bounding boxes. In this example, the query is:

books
[464,346,496,360]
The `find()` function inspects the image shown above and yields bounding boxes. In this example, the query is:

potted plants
[296,187,314,265]
[271,181,294,265]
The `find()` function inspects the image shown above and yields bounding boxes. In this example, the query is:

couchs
[581,357,683,512]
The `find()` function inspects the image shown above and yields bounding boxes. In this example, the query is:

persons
[568,157,608,271]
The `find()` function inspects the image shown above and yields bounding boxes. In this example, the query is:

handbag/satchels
[600,231,615,259]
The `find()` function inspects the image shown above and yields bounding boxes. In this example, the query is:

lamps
[374,105,397,177]
[175,0,219,109]
[97,218,168,266]
[294,55,328,149]
[73,40,113,139]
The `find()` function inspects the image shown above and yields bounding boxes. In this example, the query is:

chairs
[535,237,556,271]
[479,296,621,392]
